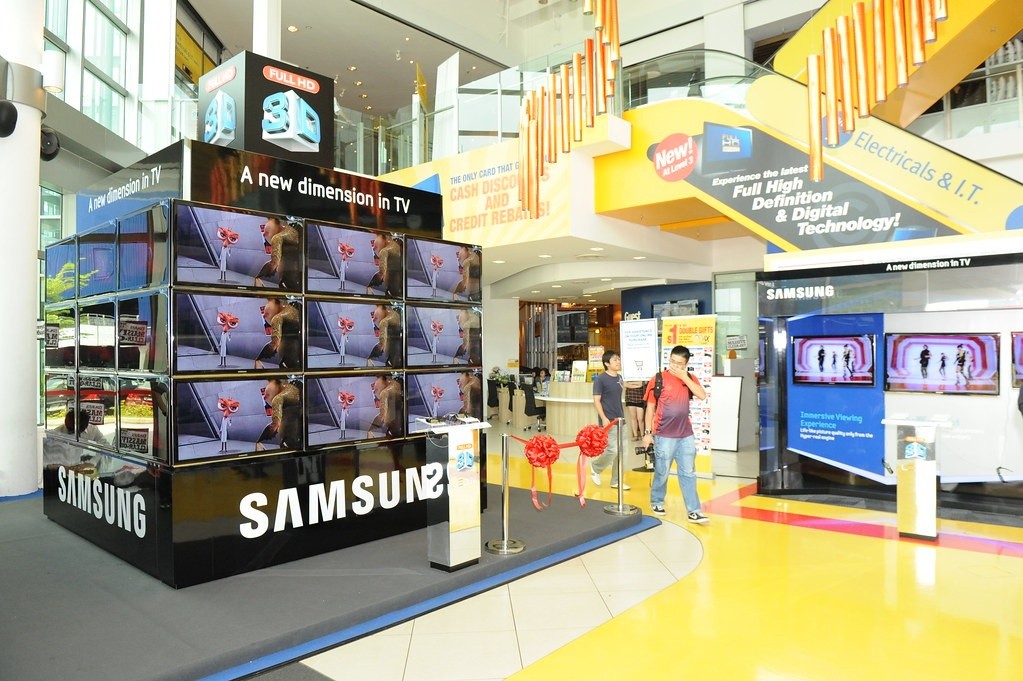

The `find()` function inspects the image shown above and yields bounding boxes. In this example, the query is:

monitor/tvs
[46,199,484,470]
[884,332,1000,395]
[1012,331,1023,388]
[790,334,876,389]
[702,121,753,175]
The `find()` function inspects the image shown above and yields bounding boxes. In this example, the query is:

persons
[952,344,971,386]
[817,344,826,373]
[967,354,975,381]
[531,367,541,386]
[623,380,647,441]
[842,344,855,378]
[850,348,857,373]
[642,344,711,524]
[936,352,948,377]
[52,408,109,446]
[591,349,632,491]
[538,368,551,382]
[919,344,931,379]
[831,351,838,370]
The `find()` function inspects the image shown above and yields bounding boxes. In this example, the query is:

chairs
[486,379,502,420]
[519,382,547,432]
[506,381,521,425]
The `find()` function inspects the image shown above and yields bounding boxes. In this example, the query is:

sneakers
[651,504,665,516]
[689,513,709,523]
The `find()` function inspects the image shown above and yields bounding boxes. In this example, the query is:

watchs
[645,428,653,435]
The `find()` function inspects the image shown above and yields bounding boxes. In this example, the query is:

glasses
[669,359,686,365]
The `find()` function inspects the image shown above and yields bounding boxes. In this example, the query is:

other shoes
[590,465,600,485]
[610,482,632,490]
